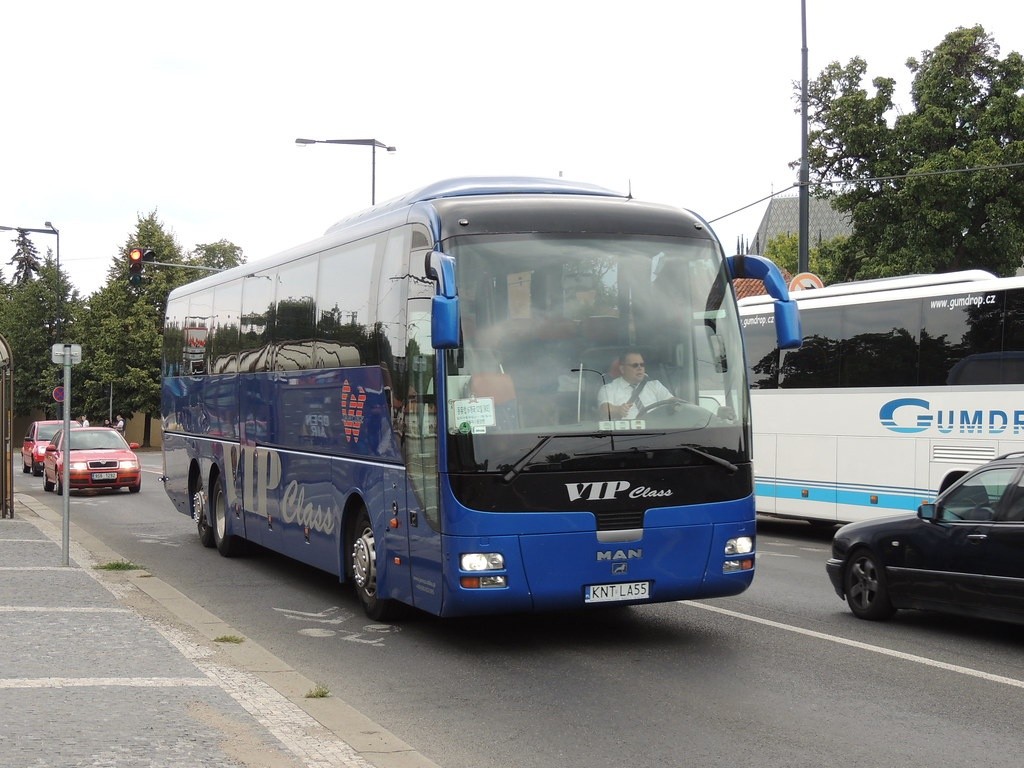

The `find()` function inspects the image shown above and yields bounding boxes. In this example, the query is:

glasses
[622,362,646,368]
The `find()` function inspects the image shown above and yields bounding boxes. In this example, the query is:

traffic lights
[130,247,155,286]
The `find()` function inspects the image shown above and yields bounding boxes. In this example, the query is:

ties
[630,383,647,416]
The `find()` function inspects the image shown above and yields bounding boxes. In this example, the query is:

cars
[23,420,83,477]
[825,452,1024,623]
[43,427,141,496]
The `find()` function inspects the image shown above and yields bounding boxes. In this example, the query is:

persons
[597,350,679,424]
[82,414,126,436]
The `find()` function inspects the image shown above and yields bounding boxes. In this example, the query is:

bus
[736,269,1023,527]
[162,178,803,620]
